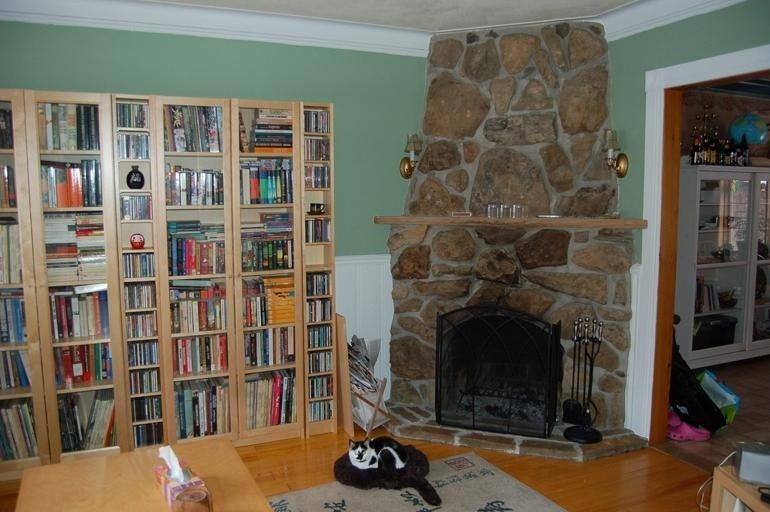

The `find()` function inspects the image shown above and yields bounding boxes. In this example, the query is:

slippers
[667,412,711,442]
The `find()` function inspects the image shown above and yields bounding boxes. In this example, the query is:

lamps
[600,127,628,178]
[398,134,424,181]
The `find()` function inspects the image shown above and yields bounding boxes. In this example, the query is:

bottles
[689,130,750,167]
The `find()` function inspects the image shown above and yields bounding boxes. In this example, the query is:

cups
[500,204,511,218]
[310,202,324,212]
[512,204,525,219]
[488,204,499,217]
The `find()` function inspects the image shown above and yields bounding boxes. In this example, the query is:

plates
[307,211,326,215]
[536,214,561,218]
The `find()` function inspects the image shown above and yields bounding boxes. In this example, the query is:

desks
[12,438,273,511]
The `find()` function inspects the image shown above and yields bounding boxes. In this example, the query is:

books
[347,334,378,392]
[703,283,719,312]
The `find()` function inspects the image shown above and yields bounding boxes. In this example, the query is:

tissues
[152,444,214,512]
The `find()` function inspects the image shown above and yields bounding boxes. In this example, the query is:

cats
[349,436,409,470]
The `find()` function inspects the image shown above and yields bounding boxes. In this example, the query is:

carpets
[265,450,567,512]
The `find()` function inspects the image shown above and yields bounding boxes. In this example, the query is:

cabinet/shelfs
[673,164,769,369]
[0,88,337,485]
[709,464,769,512]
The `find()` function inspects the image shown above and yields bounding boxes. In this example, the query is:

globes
[730,113,768,145]
